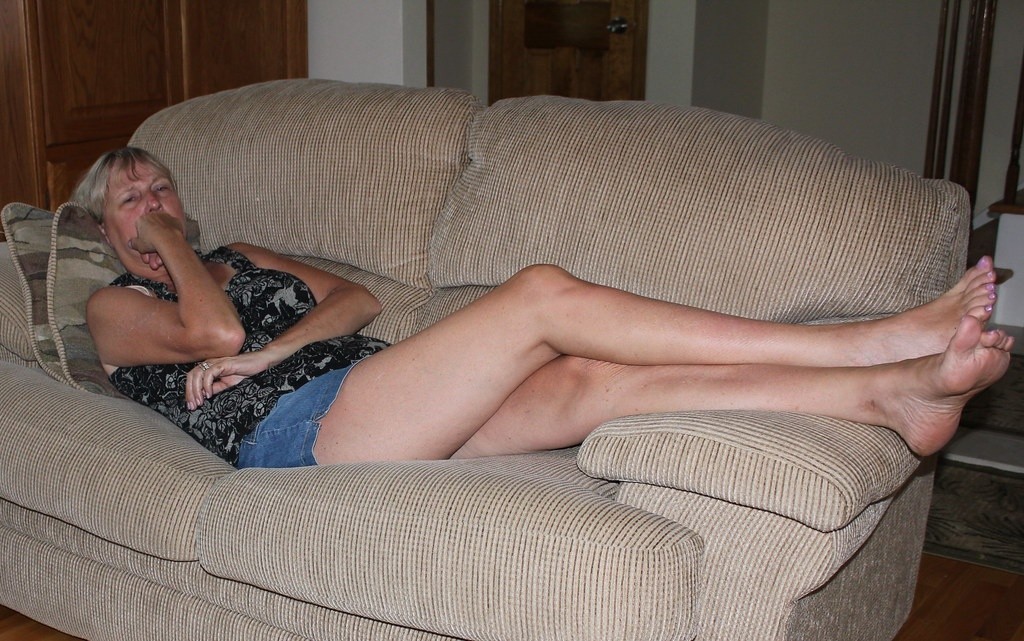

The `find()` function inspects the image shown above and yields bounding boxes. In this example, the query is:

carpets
[922,453,1024,580]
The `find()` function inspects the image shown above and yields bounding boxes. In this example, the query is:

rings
[200,361,210,371]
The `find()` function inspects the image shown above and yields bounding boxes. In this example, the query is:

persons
[69,146,1016,470]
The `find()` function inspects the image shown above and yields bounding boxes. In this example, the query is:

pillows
[2,200,201,395]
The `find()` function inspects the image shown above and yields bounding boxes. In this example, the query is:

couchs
[0,78,972,641]
